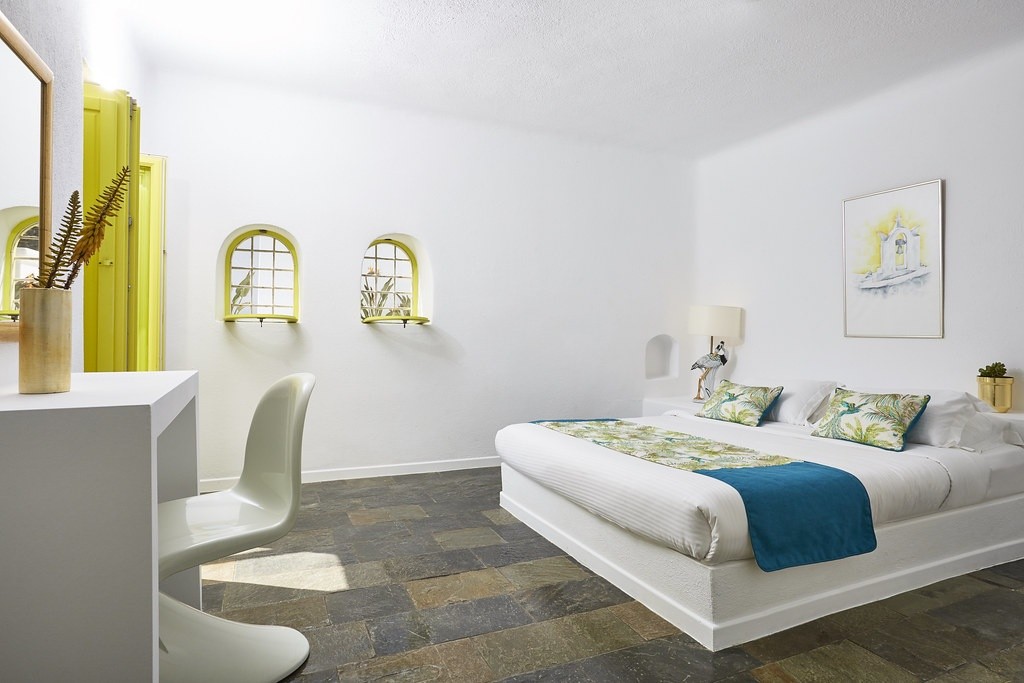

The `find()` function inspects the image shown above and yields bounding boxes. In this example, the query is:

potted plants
[20,166,130,395]
[976,361,1013,413]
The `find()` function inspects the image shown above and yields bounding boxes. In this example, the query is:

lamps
[689,304,741,402]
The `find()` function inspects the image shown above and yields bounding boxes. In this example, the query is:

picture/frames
[0,12,53,343]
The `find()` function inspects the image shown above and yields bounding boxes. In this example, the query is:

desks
[0,373,189,683]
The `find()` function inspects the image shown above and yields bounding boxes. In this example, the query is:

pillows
[885,389,1024,453]
[754,378,838,424]
[694,379,784,427]
[810,387,931,452]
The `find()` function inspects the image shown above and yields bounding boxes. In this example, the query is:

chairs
[157,371,315,683]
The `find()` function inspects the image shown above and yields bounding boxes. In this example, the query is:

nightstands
[642,396,704,419]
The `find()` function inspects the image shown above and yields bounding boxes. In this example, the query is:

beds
[494,409,1024,653]
[842,179,943,339]
